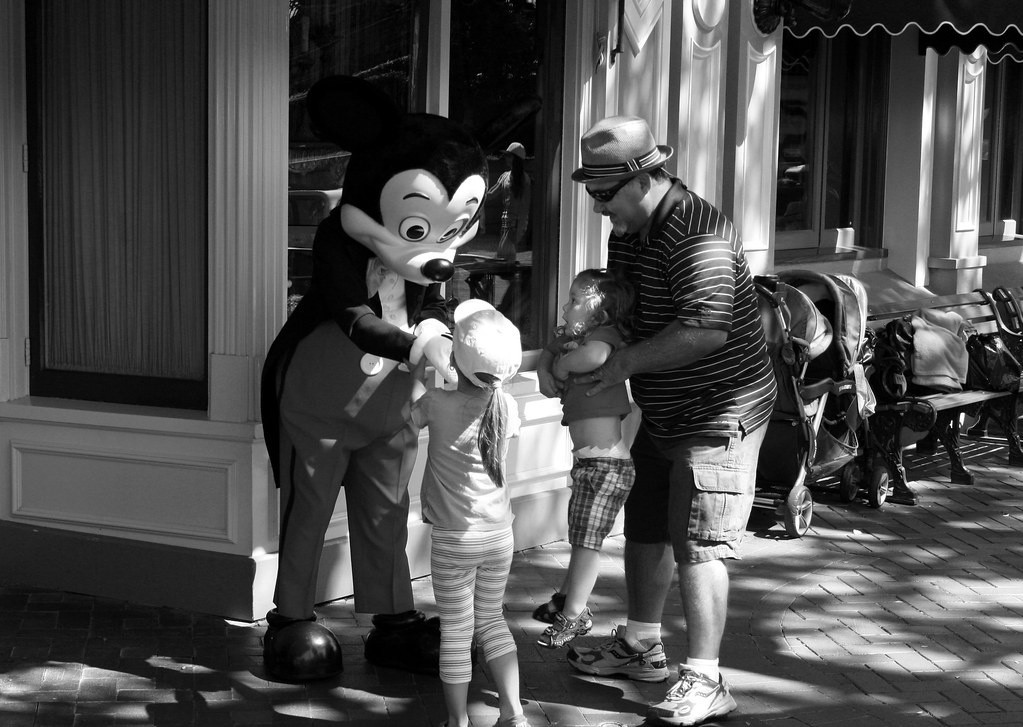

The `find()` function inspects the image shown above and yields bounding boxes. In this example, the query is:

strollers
[752,268,890,539]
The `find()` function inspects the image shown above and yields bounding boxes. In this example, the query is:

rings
[591,372,597,383]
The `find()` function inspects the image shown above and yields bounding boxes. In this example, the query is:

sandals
[532,595,564,624]
[537,607,591,649]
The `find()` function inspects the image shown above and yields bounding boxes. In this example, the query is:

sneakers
[652,663,737,726]
[561,625,670,682]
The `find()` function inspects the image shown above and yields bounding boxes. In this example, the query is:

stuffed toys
[260,74,527,684]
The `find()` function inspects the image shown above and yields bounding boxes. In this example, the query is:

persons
[536,268,638,646]
[406,299,531,727]
[556,116,780,726]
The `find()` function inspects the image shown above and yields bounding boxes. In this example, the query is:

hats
[453,297,523,389]
[501,142,525,159]
[571,115,674,185]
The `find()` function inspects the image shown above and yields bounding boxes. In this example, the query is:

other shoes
[494,716,529,727]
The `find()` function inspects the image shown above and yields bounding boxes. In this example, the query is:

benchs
[862,287,1023,506]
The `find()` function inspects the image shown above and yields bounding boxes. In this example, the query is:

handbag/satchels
[497,230,516,260]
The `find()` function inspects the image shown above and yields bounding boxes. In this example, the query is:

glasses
[585,177,635,202]
